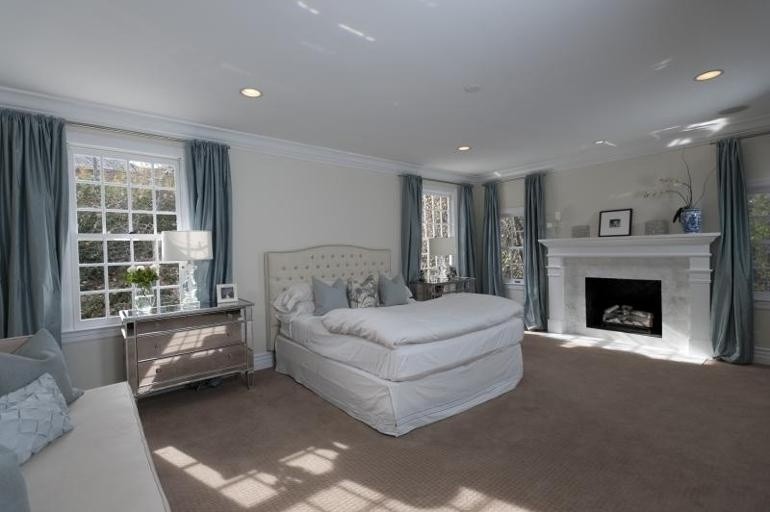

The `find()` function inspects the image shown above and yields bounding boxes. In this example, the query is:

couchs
[0,335,172,512]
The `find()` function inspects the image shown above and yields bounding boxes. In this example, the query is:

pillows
[311,273,409,316]
[1,331,83,512]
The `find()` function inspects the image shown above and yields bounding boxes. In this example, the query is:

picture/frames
[598,208,633,237]
[216,283,239,303]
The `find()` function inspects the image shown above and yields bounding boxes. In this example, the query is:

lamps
[429,236,459,278]
[161,230,214,309]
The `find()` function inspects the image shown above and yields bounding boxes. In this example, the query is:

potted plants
[126,266,161,312]
[644,148,716,234]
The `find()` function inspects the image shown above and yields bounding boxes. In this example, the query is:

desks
[118,299,255,399]
[409,276,476,301]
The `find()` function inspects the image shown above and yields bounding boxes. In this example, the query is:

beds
[264,244,524,437]
